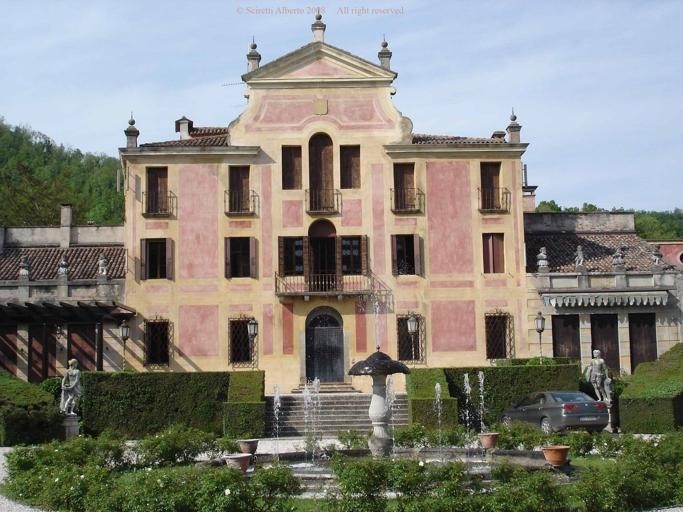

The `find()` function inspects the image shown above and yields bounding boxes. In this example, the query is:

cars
[500,390,609,437]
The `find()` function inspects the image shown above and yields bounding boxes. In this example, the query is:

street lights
[117,319,130,371]
[406,312,419,367]
[533,310,546,366]
[246,315,258,371]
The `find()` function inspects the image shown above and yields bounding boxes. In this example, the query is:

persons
[58,358,81,415]
[573,245,584,268]
[652,245,661,264]
[94,252,107,273]
[582,349,607,401]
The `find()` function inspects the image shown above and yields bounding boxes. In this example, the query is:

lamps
[338,295,343,302]
[55,320,64,339]
[303,296,310,302]
